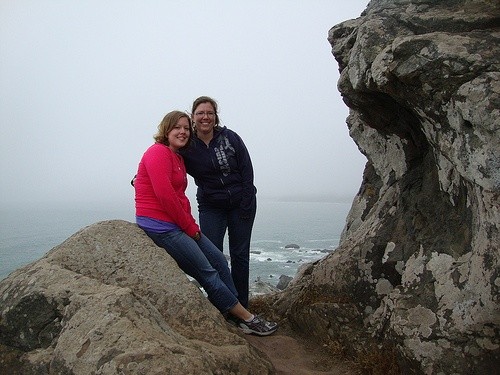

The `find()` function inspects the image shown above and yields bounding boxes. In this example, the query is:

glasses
[194,112,216,117]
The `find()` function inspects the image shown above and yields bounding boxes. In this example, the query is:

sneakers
[238,312,279,335]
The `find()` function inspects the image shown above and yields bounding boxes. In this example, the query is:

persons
[131,96,257,323]
[134,110,278,336]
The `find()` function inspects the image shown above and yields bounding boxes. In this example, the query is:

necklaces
[172,154,182,170]
